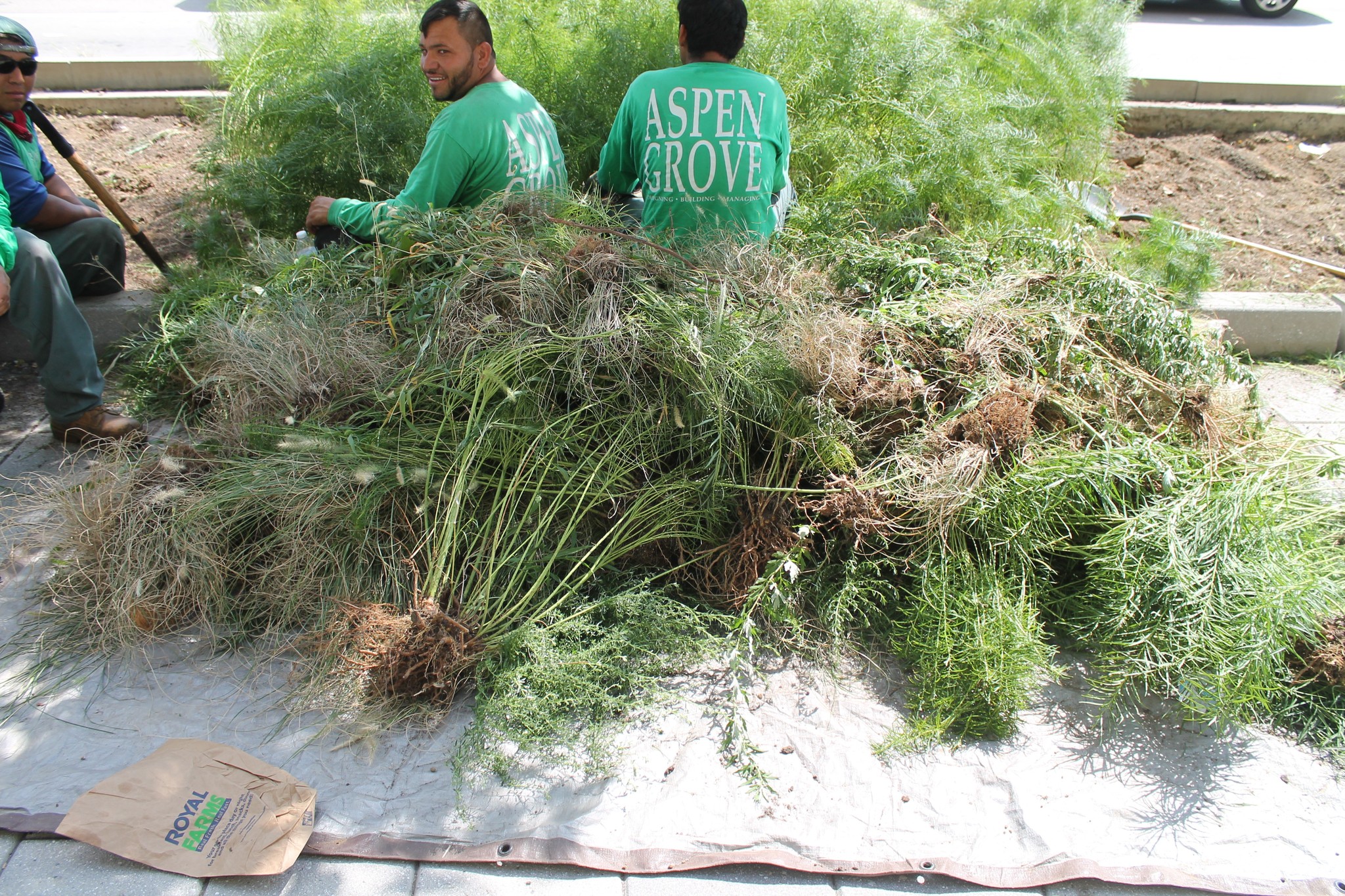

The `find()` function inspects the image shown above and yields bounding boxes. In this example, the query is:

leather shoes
[49,401,142,449]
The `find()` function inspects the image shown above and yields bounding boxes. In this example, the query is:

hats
[0,17,38,56]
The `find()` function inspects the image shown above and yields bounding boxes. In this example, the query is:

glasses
[0,56,38,76]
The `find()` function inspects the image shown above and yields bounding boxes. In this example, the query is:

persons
[0,172,149,450]
[589,0,792,265]
[0,15,126,295]
[305,0,567,258]
